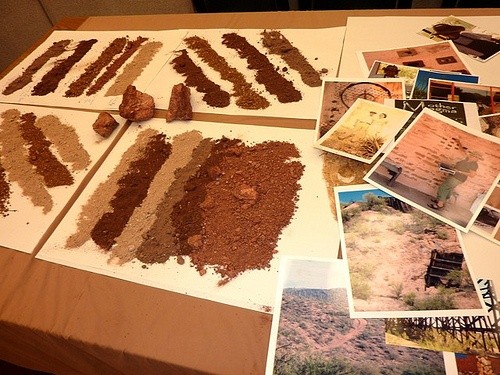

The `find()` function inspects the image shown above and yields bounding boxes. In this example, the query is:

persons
[426,151,480,210]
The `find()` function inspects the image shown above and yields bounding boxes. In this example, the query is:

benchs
[381,162,402,187]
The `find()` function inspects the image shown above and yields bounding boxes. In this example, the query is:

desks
[0,5,500,375]
